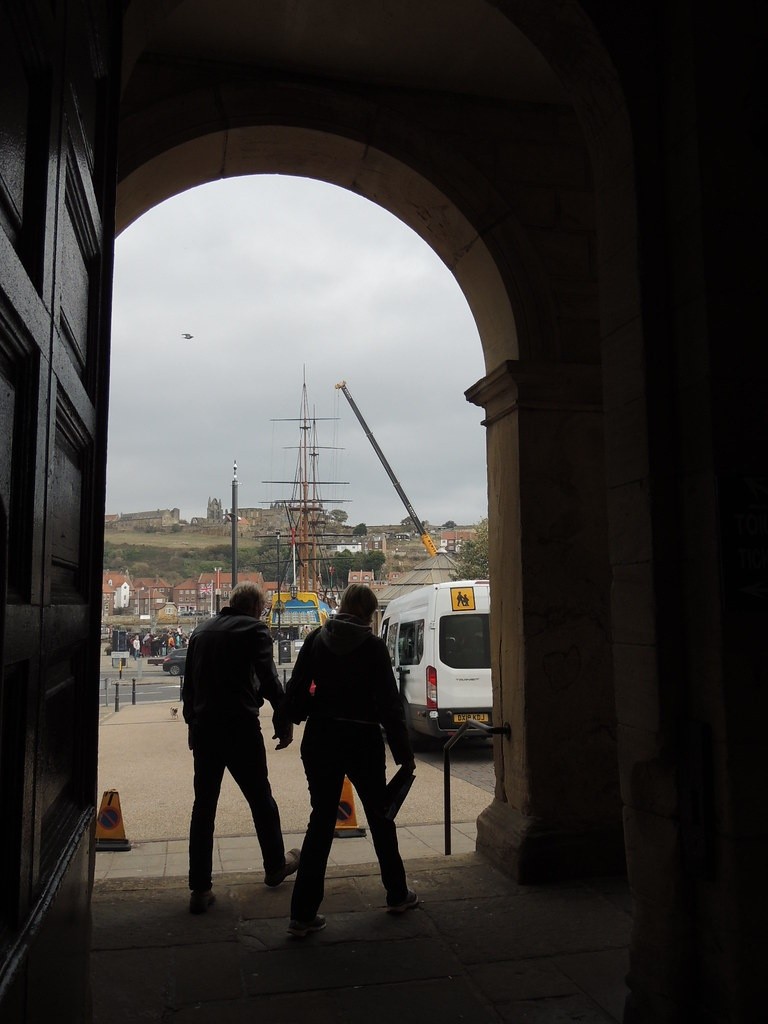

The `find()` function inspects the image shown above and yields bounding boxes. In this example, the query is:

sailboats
[252,362,362,656]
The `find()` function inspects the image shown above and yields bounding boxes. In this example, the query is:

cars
[162,648,187,676]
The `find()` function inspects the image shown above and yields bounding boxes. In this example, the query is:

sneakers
[287,913,326,936]
[386,885,419,911]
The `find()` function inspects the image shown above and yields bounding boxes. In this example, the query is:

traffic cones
[94,788,132,852]
[333,775,367,838]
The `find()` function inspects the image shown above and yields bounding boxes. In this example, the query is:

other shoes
[190,890,216,915]
[264,848,301,887]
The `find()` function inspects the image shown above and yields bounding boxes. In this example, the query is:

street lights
[274,530,281,665]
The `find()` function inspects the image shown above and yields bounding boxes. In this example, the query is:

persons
[283,584,418,936]
[127,624,192,661]
[181,582,302,914]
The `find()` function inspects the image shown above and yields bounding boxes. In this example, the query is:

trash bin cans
[280,640,291,662]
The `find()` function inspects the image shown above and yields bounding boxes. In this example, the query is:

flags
[199,583,213,596]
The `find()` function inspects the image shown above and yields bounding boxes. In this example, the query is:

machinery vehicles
[333,380,437,558]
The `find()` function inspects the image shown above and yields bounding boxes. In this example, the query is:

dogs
[170,705,178,718]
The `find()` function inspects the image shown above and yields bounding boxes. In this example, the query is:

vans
[376,579,492,754]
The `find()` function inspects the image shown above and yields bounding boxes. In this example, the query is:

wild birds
[182,333,193,339]
